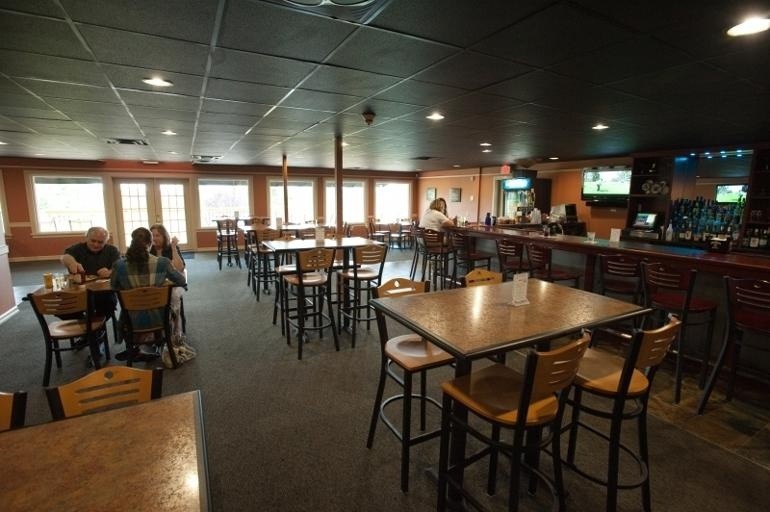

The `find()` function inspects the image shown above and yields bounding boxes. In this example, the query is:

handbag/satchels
[162,335,195,368]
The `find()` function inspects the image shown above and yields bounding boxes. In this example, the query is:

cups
[74,274,81,284]
[54,272,62,289]
[60,274,70,290]
[587,231,596,242]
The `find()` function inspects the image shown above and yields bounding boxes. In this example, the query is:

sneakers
[74,339,88,350]
[115,350,140,360]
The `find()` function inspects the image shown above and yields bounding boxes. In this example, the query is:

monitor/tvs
[581,165,633,200]
[632,212,659,229]
[715,183,749,204]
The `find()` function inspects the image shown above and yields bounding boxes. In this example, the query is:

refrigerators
[492,170,537,218]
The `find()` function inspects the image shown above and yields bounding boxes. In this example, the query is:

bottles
[749,228,759,248]
[42,272,53,289]
[484,213,497,226]
[743,229,750,247]
[543,225,557,237]
[519,188,536,207]
[759,225,767,246]
[665,195,746,243]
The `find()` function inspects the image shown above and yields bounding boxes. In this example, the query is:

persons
[110,227,186,361]
[149,223,185,343]
[416,197,456,271]
[54,227,120,353]
